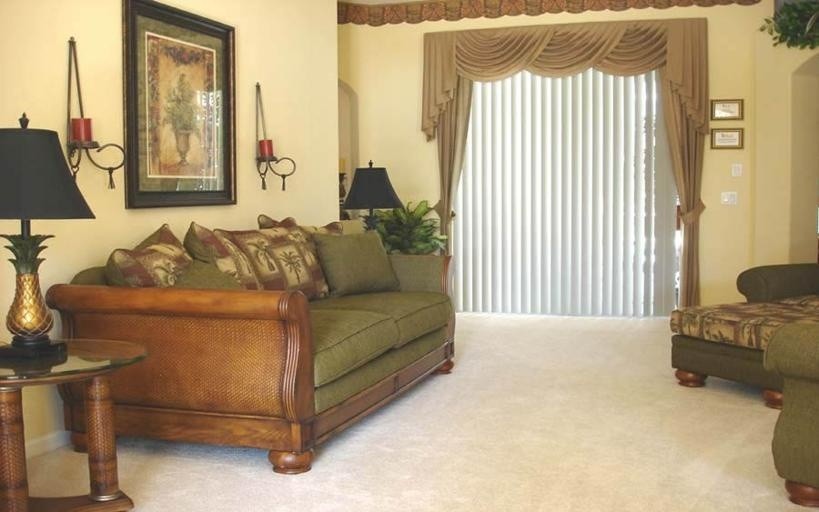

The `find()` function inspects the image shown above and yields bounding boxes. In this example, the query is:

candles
[70,115,92,143]
[258,138,274,159]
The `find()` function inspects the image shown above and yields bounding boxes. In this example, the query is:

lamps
[344,161,403,229]
[0,114,99,357]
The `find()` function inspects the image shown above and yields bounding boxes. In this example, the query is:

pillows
[311,231,401,296]
[214,226,329,301]
[259,214,296,230]
[176,258,243,290]
[183,221,266,291]
[105,222,192,288]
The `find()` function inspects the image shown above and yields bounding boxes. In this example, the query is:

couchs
[763,323,817,507]
[46,215,460,475]
[669,264,819,411]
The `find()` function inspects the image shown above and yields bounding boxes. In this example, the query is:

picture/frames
[710,128,744,150]
[120,1,239,210]
[710,98,745,121]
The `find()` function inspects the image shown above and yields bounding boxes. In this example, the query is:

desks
[0,340,147,511]
